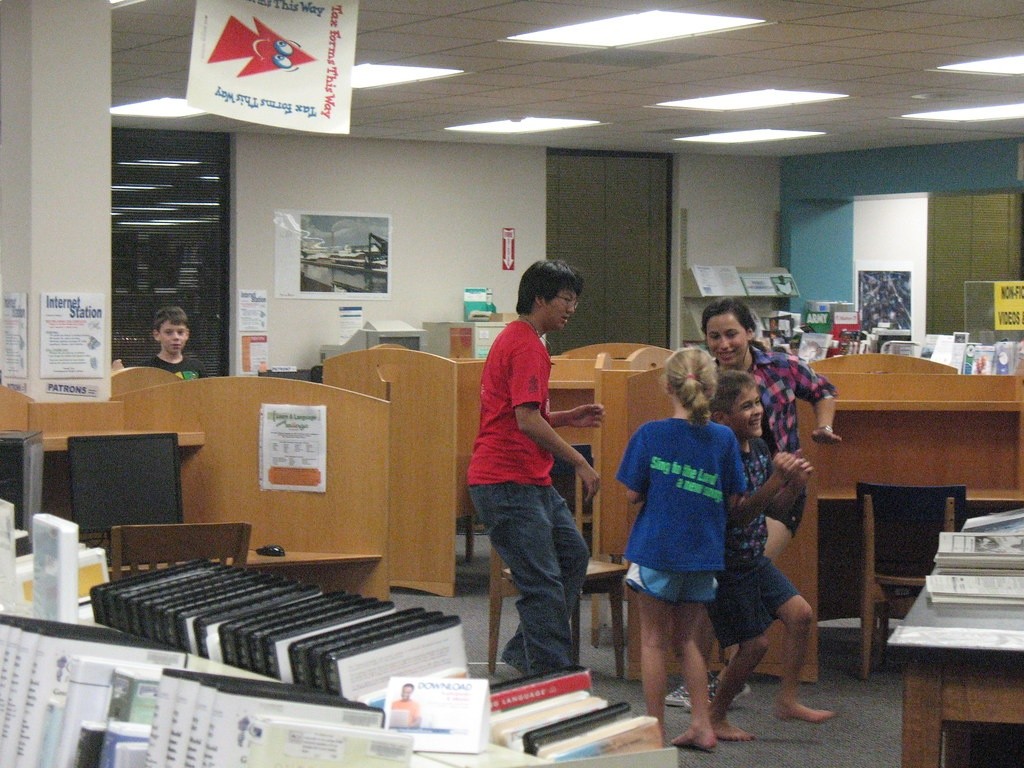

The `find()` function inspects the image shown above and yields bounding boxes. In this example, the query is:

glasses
[549,293,580,310]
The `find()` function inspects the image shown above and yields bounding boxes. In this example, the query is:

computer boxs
[1,429,43,555]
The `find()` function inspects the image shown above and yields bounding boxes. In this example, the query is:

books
[0,498,677,765]
[690,264,1023,375]
[925,531,1024,609]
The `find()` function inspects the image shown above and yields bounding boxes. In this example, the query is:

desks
[888,561,1024,768]
[105,550,381,578]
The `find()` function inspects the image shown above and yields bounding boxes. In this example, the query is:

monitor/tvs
[65,433,185,534]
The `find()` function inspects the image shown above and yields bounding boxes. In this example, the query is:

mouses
[256,544,285,556]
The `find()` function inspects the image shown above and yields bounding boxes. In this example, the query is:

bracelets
[817,424,834,433]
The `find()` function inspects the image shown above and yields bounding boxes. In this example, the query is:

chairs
[109,522,252,583]
[487,457,630,679]
[855,481,967,680]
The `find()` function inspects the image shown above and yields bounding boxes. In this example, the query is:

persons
[615,347,750,757]
[147,304,209,379]
[664,298,843,712]
[466,259,606,684]
[705,368,835,742]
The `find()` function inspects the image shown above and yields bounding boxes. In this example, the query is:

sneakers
[684,678,752,713]
[664,677,718,707]
